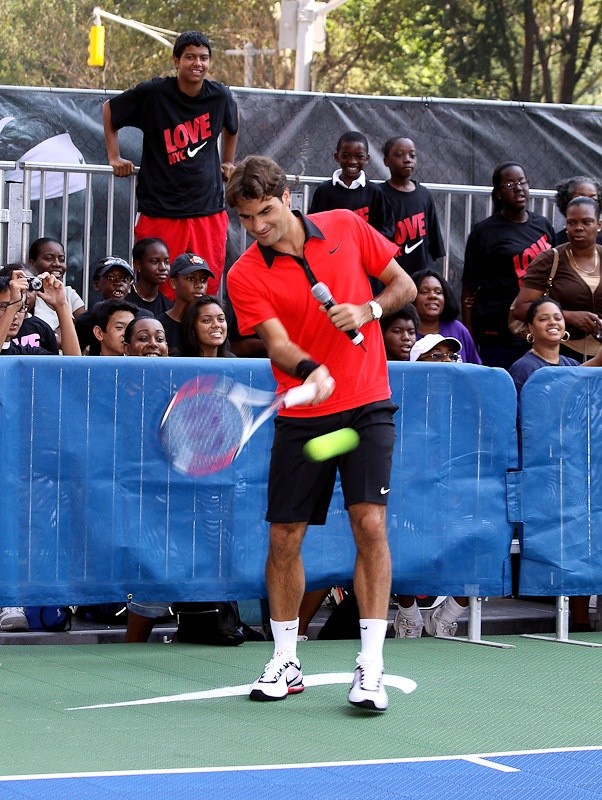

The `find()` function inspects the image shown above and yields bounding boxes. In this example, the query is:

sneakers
[393,610,425,638]
[0,607,28,630]
[249,654,304,700]
[348,653,389,711]
[423,606,457,636]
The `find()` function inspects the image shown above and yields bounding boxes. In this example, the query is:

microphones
[312,281,364,345]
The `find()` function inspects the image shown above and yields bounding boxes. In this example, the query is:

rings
[596,334,600,338]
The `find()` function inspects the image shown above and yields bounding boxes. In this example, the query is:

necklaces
[567,247,597,274]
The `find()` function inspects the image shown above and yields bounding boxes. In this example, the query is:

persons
[225,155,417,712]
[0,131,602,644]
[102,31,239,301]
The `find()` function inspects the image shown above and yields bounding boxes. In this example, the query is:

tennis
[303,426,361,462]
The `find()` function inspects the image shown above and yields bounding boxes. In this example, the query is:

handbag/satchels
[25,606,73,632]
[507,247,559,339]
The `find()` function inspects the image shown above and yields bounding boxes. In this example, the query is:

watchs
[367,300,383,321]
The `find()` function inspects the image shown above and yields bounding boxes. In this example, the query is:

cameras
[19,277,43,291]
[593,319,602,339]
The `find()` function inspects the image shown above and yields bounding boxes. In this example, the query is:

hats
[410,334,462,362]
[92,257,135,280]
[170,253,215,279]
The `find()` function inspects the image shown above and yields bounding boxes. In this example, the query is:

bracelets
[294,359,321,383]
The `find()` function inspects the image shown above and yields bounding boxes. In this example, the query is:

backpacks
[173,599,246,646]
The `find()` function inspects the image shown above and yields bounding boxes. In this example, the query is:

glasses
[498,179,528,190]
[419,351,460,362]
[0,304,29,313]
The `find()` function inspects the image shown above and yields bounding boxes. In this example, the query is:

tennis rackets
[159,375,336,477]
